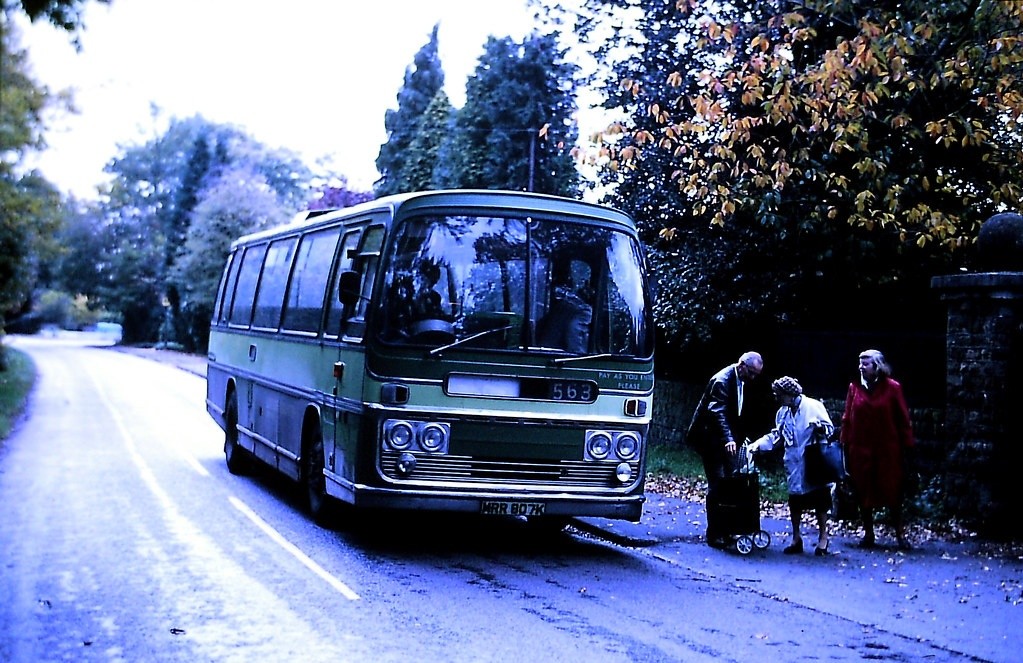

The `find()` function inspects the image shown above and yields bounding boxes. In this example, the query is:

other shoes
[896,535,911,548]
[858,532,875,549]
[707,537,738,549]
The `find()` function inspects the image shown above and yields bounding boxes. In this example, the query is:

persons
[745,376,831,556]
[686,351,764,547]
[839,349,917,549]
[538,282,597,354]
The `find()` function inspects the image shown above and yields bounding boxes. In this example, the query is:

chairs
[248,288,540,350]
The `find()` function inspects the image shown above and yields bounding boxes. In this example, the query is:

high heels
[784,540,831,557]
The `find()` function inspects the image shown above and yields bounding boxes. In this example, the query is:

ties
[737,381,743,402]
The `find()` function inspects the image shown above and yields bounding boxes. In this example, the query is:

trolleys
[712,447,769,554]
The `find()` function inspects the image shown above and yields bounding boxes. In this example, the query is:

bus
[204,187,660,540]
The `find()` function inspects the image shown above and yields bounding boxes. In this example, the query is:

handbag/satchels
[806,426,844,483]
